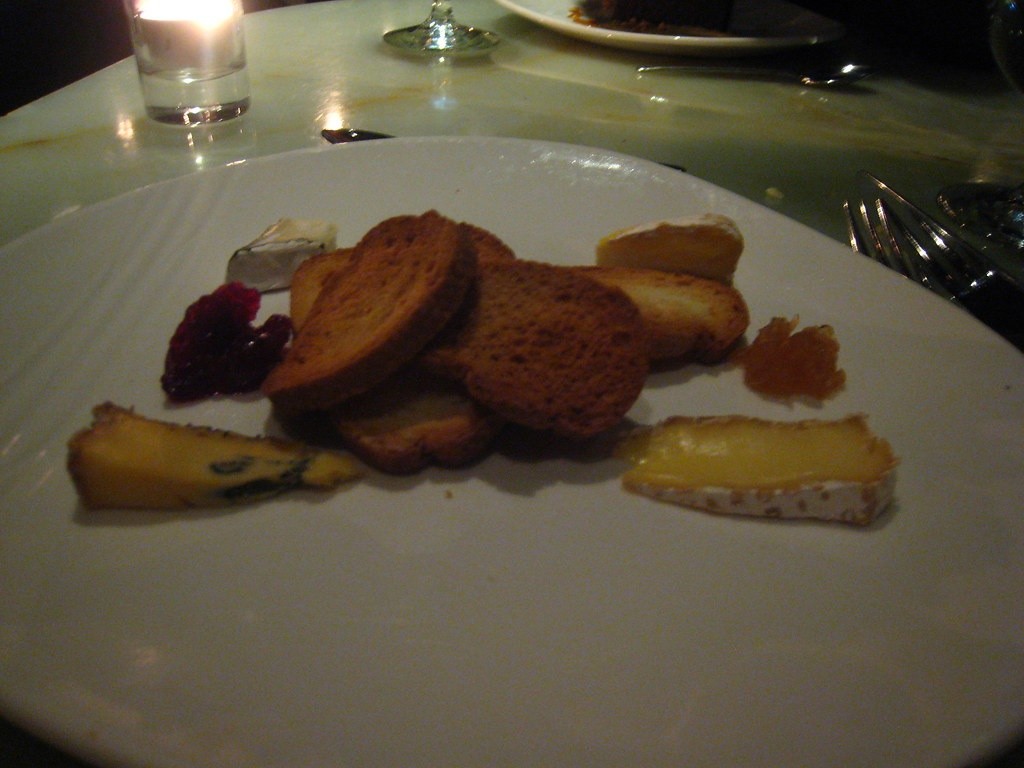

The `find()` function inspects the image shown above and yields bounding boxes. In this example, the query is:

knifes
[862,169,1023,294]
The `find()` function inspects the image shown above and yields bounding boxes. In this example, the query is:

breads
[265,210,751,473]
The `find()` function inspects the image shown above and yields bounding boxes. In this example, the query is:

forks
[841,198,911,281]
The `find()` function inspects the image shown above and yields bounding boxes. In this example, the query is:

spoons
[635,59,877,88]
[322,126,686,172]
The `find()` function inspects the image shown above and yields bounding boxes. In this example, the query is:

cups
[122,0,252,125]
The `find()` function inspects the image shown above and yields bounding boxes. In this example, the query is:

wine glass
[382,0,501,56]
[935,0,1024,253]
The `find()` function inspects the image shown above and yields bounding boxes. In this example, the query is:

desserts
[615,413,898,525]
[66,400,357,508]
[595,214,744,285]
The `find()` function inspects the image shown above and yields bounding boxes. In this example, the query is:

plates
[0,135,1024,766]
[496,0,831,58]
